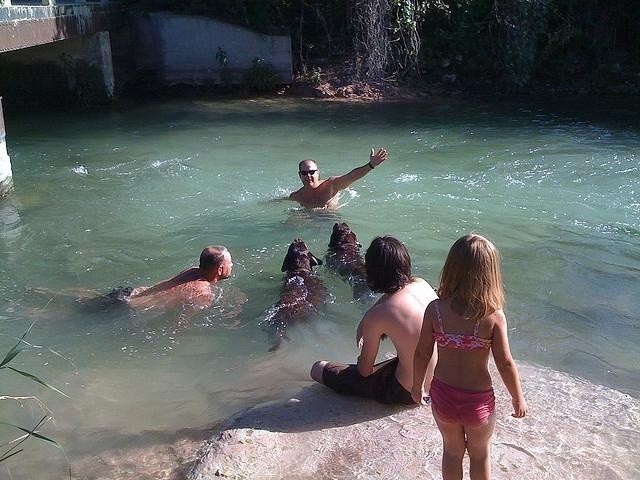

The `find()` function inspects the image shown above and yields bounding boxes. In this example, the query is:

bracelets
[368,162,375,169]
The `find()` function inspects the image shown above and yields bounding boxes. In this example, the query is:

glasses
[301,170,318,176]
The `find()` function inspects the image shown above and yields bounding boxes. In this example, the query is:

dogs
[322,223,376,315]
[263,239,327,351]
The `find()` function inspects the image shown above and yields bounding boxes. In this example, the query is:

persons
[100,245,234,321]
[310,235,439,406]
[410,234,528,480]
[289,147,388,210]
